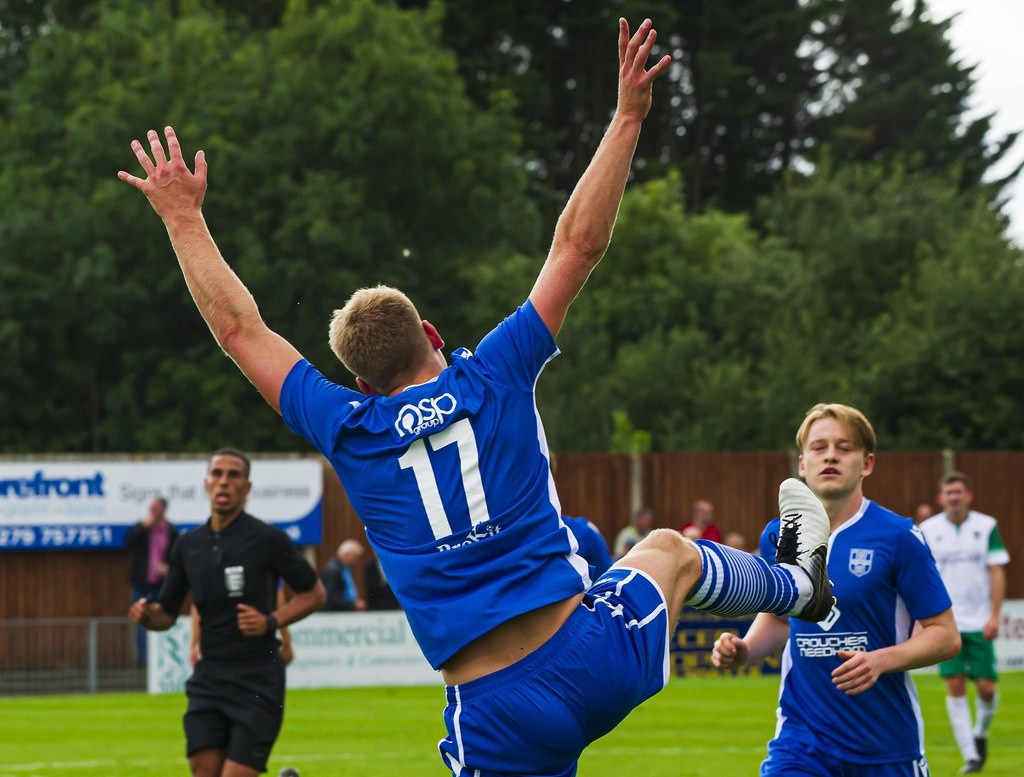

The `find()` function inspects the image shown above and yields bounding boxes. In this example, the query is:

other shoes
[958,758,983,774]
[974,737,986,763]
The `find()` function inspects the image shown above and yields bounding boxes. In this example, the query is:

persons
[549,453,613,586]
[320,539,377,613]
[679,499,719,543]
[117,14,833,777]
[918,472,1010,773]
[124,499,180,670]
[724,532,747,551]
[712,402,964,777]
[129,448,327,777]
[615,506,655,557]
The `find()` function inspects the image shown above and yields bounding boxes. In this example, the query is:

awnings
[0,457,324,550]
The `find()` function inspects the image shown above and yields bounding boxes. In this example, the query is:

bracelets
[265,611,277,633]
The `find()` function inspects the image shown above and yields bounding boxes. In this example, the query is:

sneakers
[768,477,833,623]
